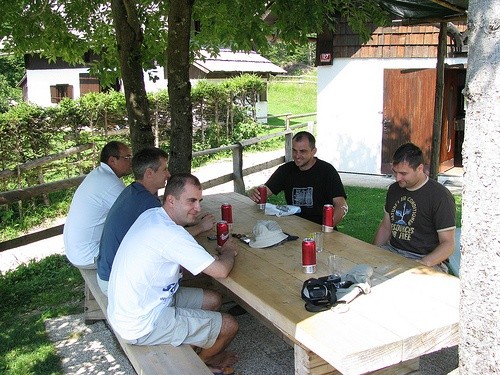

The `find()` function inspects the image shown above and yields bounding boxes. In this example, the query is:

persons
[373,144,456,274]
[97,147,216,296]
[107,173,240,374]
[63,141,132,270]
[247,131,348,231]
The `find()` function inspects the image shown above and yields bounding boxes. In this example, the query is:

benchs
[80,269,215,375]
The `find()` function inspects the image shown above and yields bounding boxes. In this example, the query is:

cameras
[307,281,330,301]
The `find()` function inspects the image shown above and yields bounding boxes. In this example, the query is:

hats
[249,220,288,248]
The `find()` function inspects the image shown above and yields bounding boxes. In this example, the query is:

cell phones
[207,234,217,240]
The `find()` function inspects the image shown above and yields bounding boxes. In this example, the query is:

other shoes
[229,304,247,315]
[207,367,234,375]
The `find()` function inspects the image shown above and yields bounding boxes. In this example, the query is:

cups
[310,231,324,252]
[327,254,343,277]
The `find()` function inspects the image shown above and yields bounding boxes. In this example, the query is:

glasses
[112,156,133,162]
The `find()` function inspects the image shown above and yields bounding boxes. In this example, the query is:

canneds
[321,204,333,233]
[302,238,316,273]
[257,184,267,209]
[220,203,233,229]
[217,221,229,247]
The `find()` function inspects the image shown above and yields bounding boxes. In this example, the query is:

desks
[195,191,460,375]
